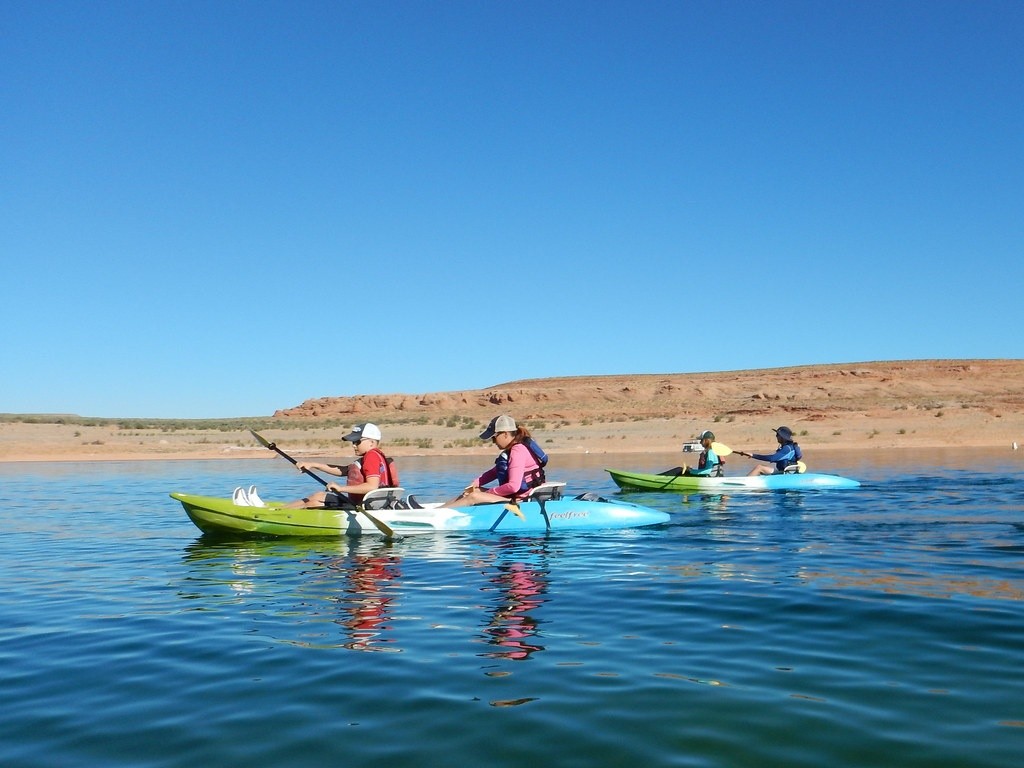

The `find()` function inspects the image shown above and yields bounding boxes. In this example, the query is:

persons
[657,429,723,477]
[406,414,553,509]
[233,420,401,509]
[745,426,797,477]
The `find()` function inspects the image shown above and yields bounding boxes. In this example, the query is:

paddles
[682,463,692,475]
[504,498,526,521]
[246,424,393,538]
[711,441,806,474]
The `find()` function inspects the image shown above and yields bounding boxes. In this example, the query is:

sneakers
[393,500,408,510]
[407,495,424,509]
[248,485,264,507]
[232,486,250,506]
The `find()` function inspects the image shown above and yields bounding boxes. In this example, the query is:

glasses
[352,437,370,445]
[491,433,501,439]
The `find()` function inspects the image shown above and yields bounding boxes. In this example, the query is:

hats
[342,423,381,441]
[696,431,715,439]
[479,415,517,439]
[772,426,793,442]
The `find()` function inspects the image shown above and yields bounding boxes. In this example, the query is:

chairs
[707,463,724,476]
[784,464,799,474]
[527,482,568,502]
[362,487,405,508]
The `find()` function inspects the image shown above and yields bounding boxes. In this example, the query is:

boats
[604,467,864,492]
[170,490,673,533]
